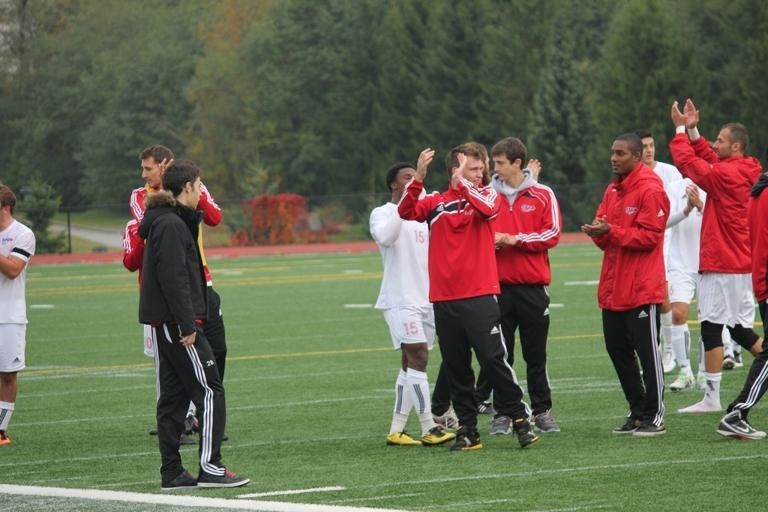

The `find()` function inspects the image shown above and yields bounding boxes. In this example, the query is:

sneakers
[192,415,229,442]
[662,349,676,373]
[669,370,697,391]
[490,416,513,435]
[387,431,422,446]
[161,470,198,490]
[514,418,539,448]
[716,412,766,440]
[677,401,721,413]
[0,430,10,446]
[180,434,196,445]
[149,420,193,435]
[722,355,735,369]
[422,428,456,446]
[613,416,643,434]
[478,401,493,414]
[197,468,250,487]
[450,427,482,451]
[633,421,666,437]
[734,350,743,367]
[533,409,560,432]
[432,409,460,429]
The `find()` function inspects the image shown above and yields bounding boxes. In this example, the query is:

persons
[121,217,200,436]
[137,160,252,492]
[425,143,543,432]
[668,97,763,415]
[395,140,540,451]
[720,324,745,370]
[0,182,39,446]
[635,129,684,376]
[665,175,708,393]
[484,136,563,436]
[127,143,230,446]
[368,164,459,447]
[715,170,768,441]
[578,130,672,437]
